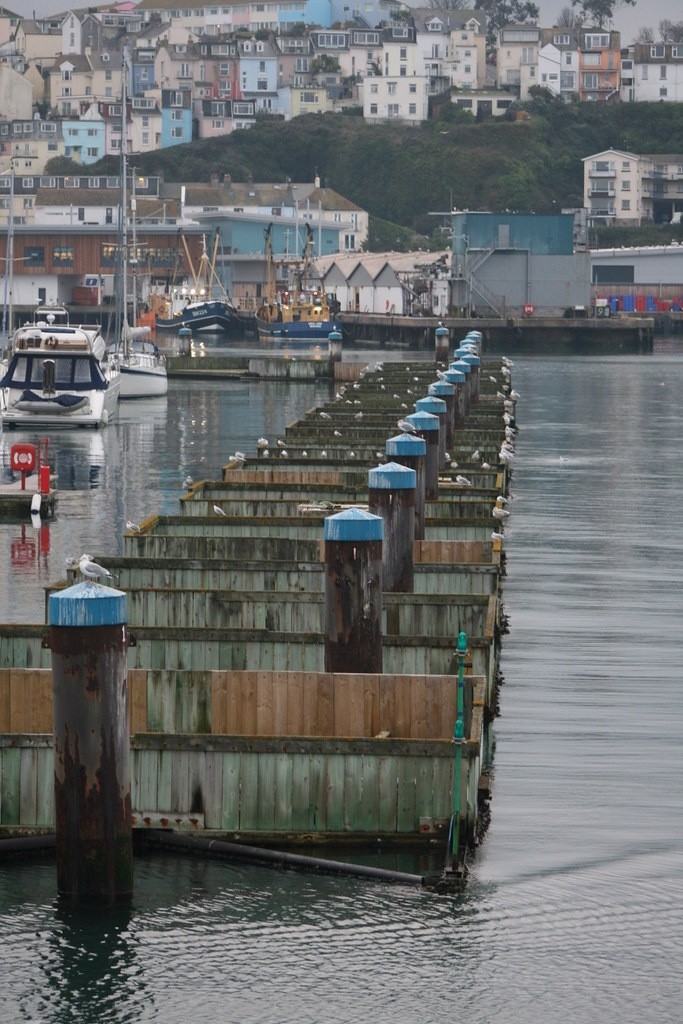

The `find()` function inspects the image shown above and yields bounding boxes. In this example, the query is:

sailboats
[103,168,168,400]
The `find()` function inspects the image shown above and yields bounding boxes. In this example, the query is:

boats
[154,226,239,344]
[253,222,344,347]
[0,304,121,430]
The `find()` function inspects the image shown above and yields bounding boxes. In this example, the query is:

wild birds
[353,412,363,422]
[350,451,355,461]
[429,384,437,397]
[491,495,510,543]
[465,346,478,356]
[435,360,449,382]
[375,450,385,460]
[229,452,247,464]
[396,420,419,435]
[438,321,448,329]
[257,437,288,459]
[212,504,227,516]
[444,448,492,488]
[334,430,344,439]
[488,375,498,384]
[66,555,118,585]
[182,476,195,489]
[358,360,385,390]
[321,451,327,459]
[125,520,144,534]
[319,412,332,421]
[302,450,308,458]
[335,380,362,405]
[392,366,419,409]
[497,357,521,467]
